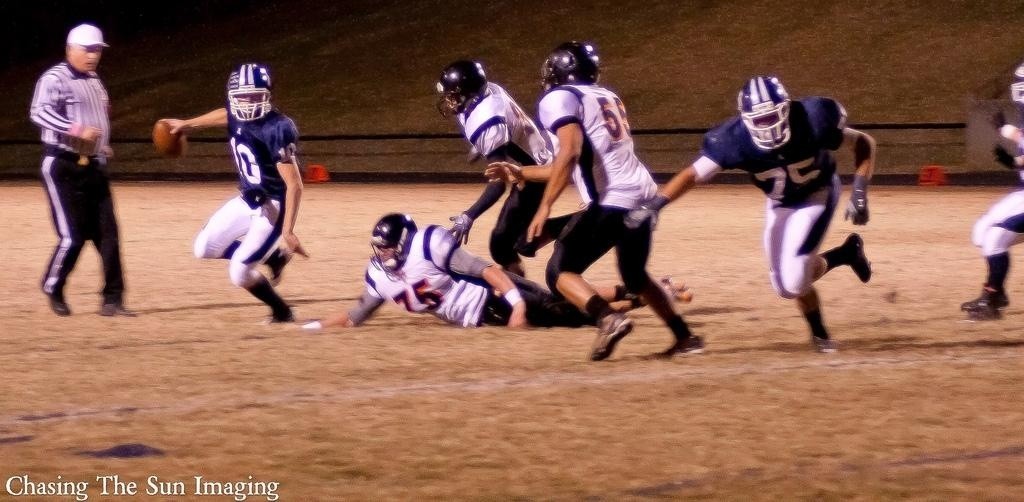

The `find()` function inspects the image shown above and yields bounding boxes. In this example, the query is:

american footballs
[152,119,181,160]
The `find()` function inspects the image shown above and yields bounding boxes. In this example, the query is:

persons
[622,75,877,346]
[300,215,694,335]
[437,55,586,277]
[485,39,707,363]
[28,24,135,317]
[156,62,309,328]
[961,61,1024,320]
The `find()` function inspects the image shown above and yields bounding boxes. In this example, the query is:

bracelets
[502,288,522,303]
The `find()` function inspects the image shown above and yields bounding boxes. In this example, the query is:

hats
[67,24,109,49]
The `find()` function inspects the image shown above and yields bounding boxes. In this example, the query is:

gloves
[447,213,474,246]
[845,175,869,225]
[990,111,1006,128]
[990,144,1015,169]
[624,193,670,229]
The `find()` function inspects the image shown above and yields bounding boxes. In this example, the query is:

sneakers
[100,304,136,317]
[46,290,71,316]
[591,314,633,361]
[661,278,692,302]
[269,249,293,287]
[846,233,871,282]
[660,337,704,358]
[960,284,1008,310]
[813,331,837,353]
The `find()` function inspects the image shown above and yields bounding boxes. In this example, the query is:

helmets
[435,60,487,118]
[226,64,272,122]
[371,214,418,280]
[540,41,600,90]
[738,77,792,150]
[1010,64,1024,102]
